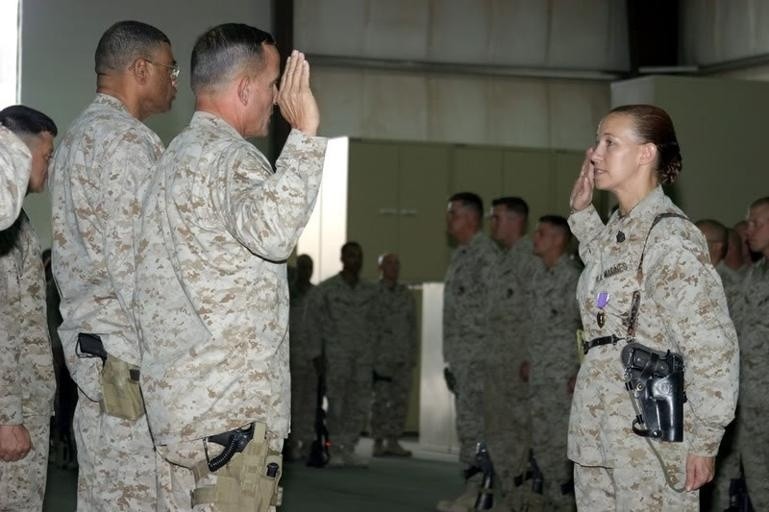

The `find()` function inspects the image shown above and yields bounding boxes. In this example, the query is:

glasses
[129,58,180,81]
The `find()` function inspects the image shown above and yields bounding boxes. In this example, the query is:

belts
[582,336,627,353]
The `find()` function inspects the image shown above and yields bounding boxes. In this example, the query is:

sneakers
[438,477,482,512]
[345,450,372,466]
[300,445,328,462]
[369,440,385,455]
[281,447,299,463]
[385,439,411,456]
[323,449,352,468]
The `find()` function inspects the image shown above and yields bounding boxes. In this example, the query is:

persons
[0,105,59,511]
[0,122,34,231]
[567,103,740,511]
[695,197,769,512]
[132,23,327,511]
[48,19,180,510]
[289,241,414,467]
[440,192,586,511]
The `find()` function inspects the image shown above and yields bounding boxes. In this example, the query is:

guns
[203,422,254,472]
[75,331,107,366]
[724,454,752,512]
[514,448,542,494]
[315,372,331,468]
[561,480,574,494]
[464,441,492,510]
[623,343,687,442]
